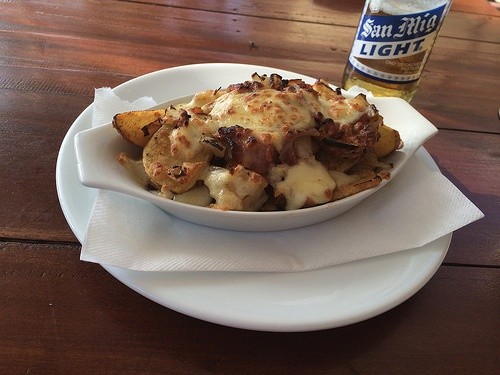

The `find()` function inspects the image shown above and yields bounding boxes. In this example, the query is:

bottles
[340,0,453,103]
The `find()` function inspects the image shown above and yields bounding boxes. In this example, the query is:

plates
[55,62,452,333]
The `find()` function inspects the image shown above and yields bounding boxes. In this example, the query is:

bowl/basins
[74,88,438,232]
